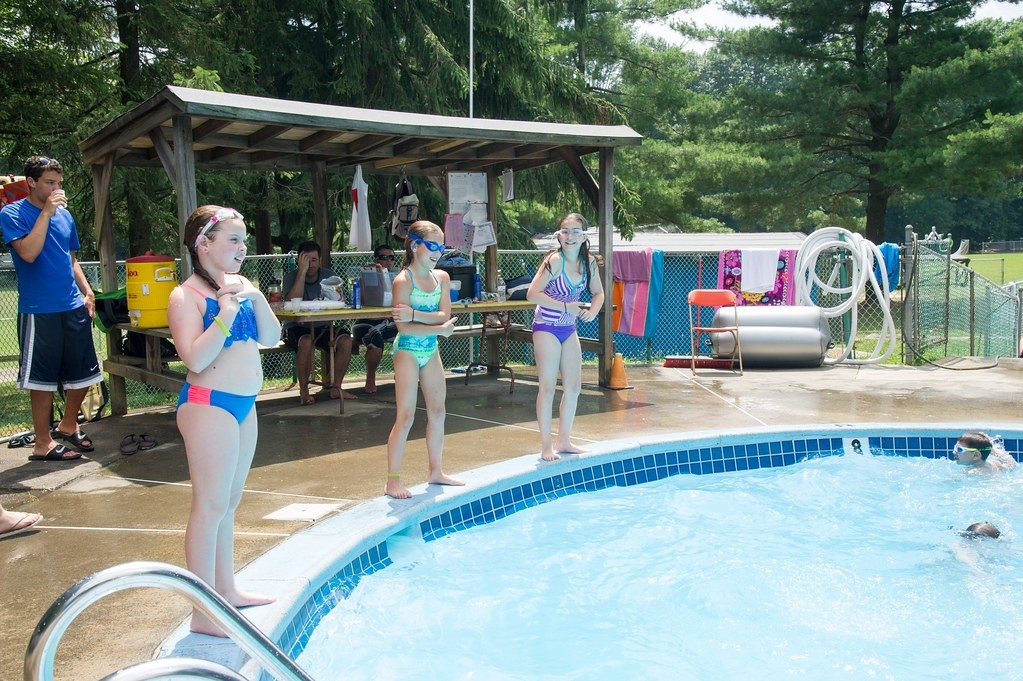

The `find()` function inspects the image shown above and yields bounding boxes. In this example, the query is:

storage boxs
[362,272,398,308]
[434,265,478,301]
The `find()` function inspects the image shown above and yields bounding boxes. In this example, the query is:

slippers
[120,433,139,455]
[139,433,157,449]
[55,427,95,451]
[0,512,43,538]
[28,444,82,460]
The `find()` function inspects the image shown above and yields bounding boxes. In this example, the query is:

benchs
[113,323,524,365]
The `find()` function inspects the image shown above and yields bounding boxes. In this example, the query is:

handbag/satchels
[90,286,131,333]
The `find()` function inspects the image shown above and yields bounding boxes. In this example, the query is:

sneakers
[500,313,511,326]
[486,315,502,327]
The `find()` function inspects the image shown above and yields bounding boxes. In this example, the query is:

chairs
[688,289,744,376]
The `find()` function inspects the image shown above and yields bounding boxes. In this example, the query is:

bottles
[352,277,361,309]
[267,279,282,310]
[473,260,482,302]
[496,270,506,304]
[91,241,99,261]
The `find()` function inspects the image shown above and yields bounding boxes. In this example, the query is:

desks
[274,300,540,416]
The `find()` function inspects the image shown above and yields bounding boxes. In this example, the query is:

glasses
[559,229,585,237]
[376,255,394,260]
[194,208,244,247]
[31,157,59,174]
[425,241,446,254]
[953,445,978,454]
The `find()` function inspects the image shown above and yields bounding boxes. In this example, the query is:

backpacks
[389,174,421,242]
[116,330,178,358]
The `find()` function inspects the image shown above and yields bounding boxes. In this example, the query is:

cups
[50,189,67,209]
[291,297,302,312]
[284,301,346,310]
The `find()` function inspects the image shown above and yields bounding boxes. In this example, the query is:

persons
[0,158,104,460]
[167,205,281,638]
[953,432,1015,476]
[968,523,1000,539]
[355,245,397,393]
[385,220,465,498]
[0,505,43,538]
[526,213,604,461]
[283,242,358,404]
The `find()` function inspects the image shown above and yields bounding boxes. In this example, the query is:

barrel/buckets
[126,249,179,328]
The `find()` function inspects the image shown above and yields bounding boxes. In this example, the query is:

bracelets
[564,302,567,314]
[215,316,231,337]
[412,309,415,321]
